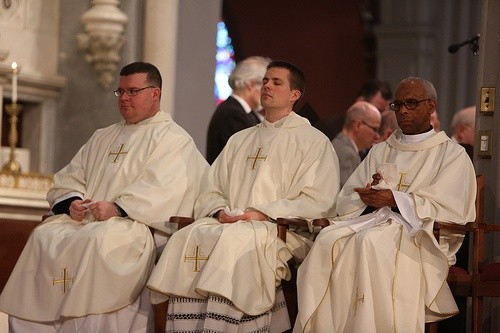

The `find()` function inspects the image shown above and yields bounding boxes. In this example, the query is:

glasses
[389,99,431,111]
[361,121,379,132]
[114,86,156,97]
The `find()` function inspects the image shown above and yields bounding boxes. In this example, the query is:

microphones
[449,34,480,53]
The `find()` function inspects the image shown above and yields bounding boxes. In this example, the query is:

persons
[293,77,477,333]
[146,60,341,333]
[450,106,476,162]
[206,56,272,166]
[359,76,398,161]
[0,61,211,333]
[332,102,382,190]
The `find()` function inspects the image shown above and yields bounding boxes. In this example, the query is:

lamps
[76,0,130,91]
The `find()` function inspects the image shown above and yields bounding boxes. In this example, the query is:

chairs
[41,175,500,333]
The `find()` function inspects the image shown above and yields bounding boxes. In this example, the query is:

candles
[11,61,18,103]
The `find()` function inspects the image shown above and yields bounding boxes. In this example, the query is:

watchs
[116,208,121,215]
